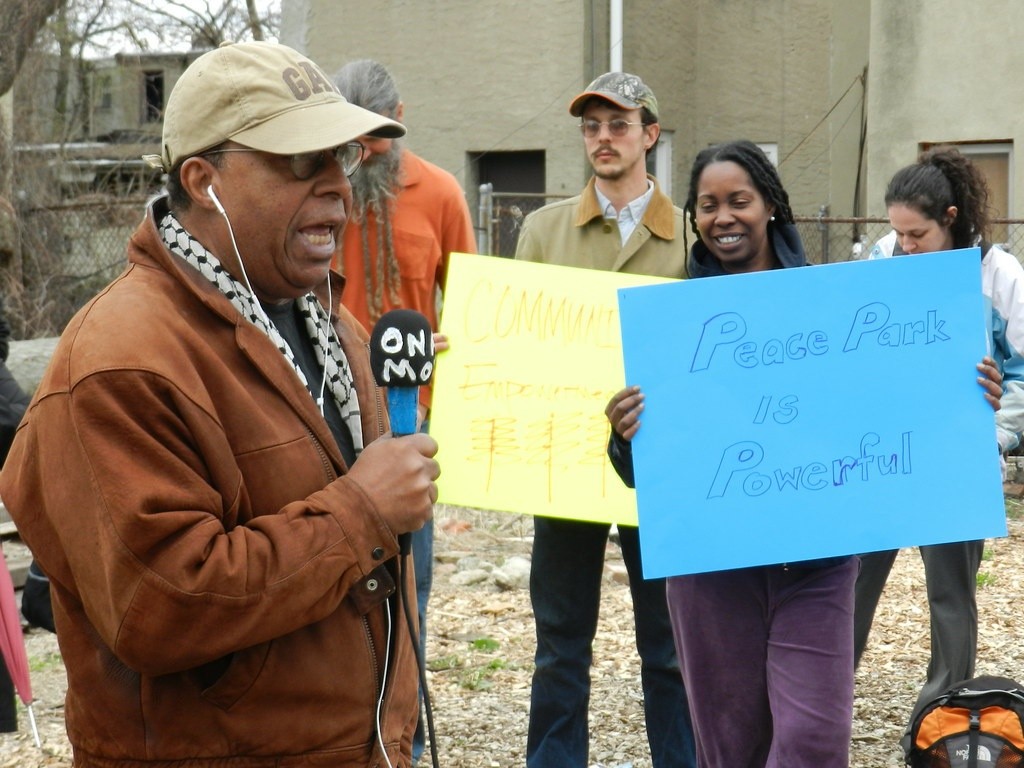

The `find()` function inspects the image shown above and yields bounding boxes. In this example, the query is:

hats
[161,40,407,175]
[569,71,659,117]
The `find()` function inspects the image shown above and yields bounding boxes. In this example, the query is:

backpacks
[900,675,1024,768]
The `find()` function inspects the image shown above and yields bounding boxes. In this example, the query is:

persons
[0,42,475,768]
[434,73,696,768]
[605,142,1024,768]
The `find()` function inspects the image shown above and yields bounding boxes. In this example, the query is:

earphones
[207,185,225,213]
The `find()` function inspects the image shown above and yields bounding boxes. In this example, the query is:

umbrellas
[0,549,41,749]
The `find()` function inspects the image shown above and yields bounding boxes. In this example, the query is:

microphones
[371,309,436,555]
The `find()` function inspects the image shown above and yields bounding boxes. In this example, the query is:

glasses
[199,140,366,180]
[578,119,646,137]
